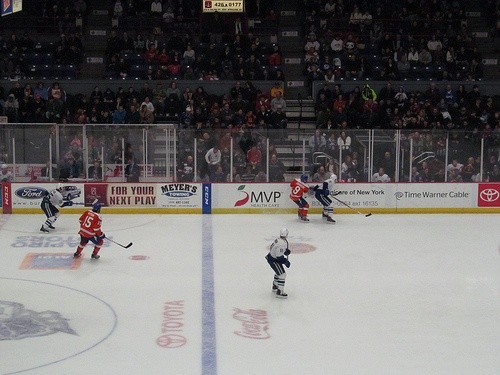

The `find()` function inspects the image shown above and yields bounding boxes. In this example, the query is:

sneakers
[48,225,55,230]
[301,217,309,222]
[272,286,281,292]
[91,253,100,258]
[276,291,288,298]
[323,213,329,218]
[40,227,49,233]
[74,252,81,257]
[327,217,337,224]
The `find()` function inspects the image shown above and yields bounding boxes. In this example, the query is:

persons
[0,0,500,183]
[314,172,337,223]
[265,228,290,298]
[290,174,311,221]
[40,182,73,233]
[73,203,104,259]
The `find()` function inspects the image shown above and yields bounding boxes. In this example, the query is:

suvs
[57,186,81,200]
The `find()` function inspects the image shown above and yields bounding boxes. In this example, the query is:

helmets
[330,175,338,181]
[92,204,101,211]
[301,174,309,181]
[280,228,288,237]
[57,185,65,194]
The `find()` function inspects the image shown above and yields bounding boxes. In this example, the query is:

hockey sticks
[285,238,289,261]
[103,235,133,249]
[67,198,99,206]
[331,194,372,217]
[312,186,342,196]
